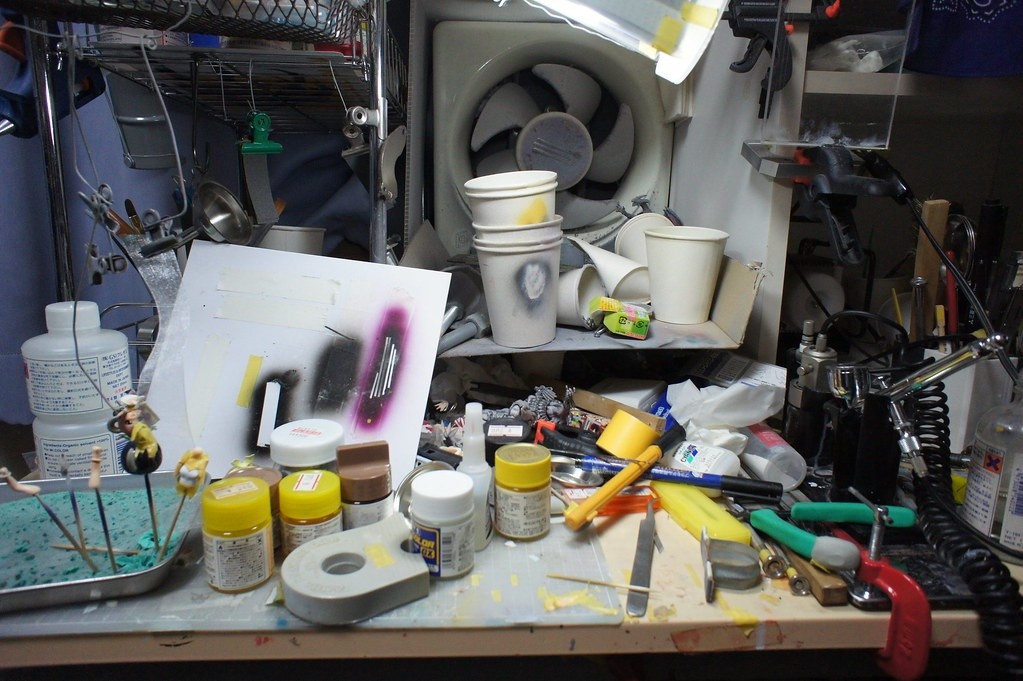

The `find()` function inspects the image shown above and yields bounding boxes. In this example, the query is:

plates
[551,454,604,488]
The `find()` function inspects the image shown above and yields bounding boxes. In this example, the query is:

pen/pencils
[580,454,784,506]
[565,423,687,531]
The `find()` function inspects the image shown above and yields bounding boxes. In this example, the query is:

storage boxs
[921,349,1019,455]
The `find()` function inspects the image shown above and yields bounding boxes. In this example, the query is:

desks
[0,467,1023,669]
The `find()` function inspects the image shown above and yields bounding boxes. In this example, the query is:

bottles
[962,368,1023,567]
[738,420,807,488]
[21,300,132,480]
[415,402,552,580]
[336,441,394,530]
[202,419,343,594]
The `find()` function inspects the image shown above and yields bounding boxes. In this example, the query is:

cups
[464,170,564,349]
[558,214,729,331]
[249,224,324,256]
